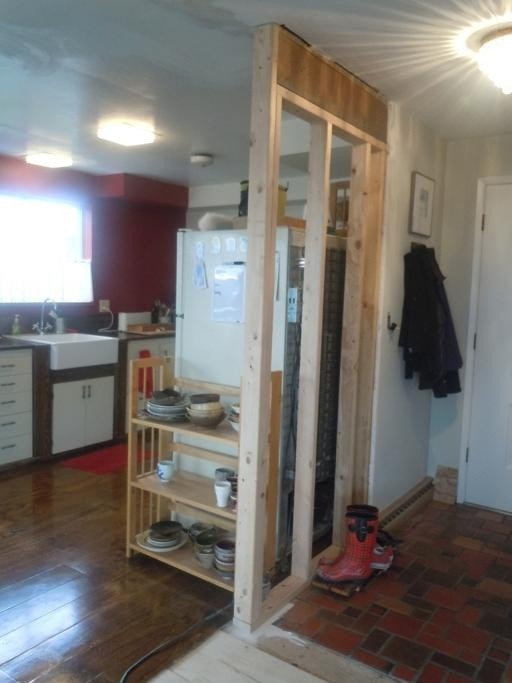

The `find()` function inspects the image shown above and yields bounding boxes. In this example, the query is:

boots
[317,505,379,584]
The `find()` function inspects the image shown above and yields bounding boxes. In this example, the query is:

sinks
[5,331,119,371]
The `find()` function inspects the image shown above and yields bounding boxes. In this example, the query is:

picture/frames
[408,169,436,238]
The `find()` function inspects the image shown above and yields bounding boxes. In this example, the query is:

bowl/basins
[182,393,227,429]
[195,530,234,582]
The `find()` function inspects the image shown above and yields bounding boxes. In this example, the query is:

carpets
[56,443,159,476]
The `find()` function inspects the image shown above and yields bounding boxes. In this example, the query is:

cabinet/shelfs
[124,354,281,588]
[50,375,115,455]
[124,336,175,435]
[0,348,36,464]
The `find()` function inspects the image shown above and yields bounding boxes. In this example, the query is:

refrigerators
[174,226,345,562]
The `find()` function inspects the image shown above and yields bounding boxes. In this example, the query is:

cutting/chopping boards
[128,324,175,332]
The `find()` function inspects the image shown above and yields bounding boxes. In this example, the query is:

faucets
[40,298,58,331]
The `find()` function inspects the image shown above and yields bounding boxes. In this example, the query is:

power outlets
[97,299,109,312]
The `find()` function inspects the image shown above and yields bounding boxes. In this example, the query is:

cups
[54,317,64,333]
[215,469,231,506]
[157,461,173,483]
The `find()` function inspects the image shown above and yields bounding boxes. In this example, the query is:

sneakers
[370,529,403,569]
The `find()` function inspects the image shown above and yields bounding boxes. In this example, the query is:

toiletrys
[12,312,24,333]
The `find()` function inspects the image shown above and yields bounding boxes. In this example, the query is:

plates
[143,391,187,423]
[134,521,188,553]
